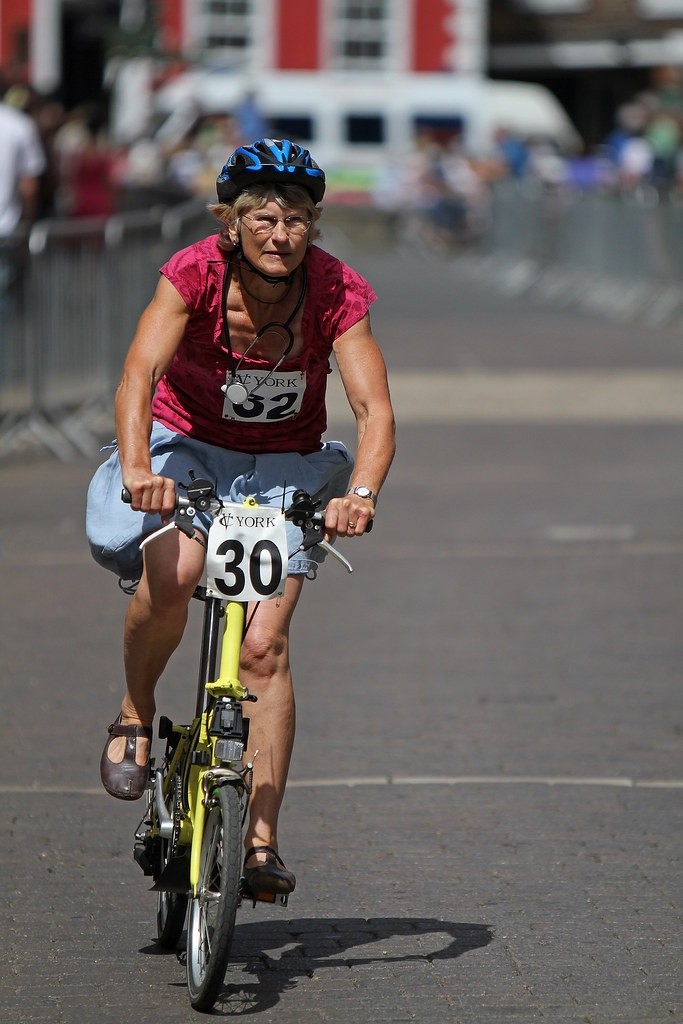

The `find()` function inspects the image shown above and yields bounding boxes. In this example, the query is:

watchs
[345,486,377,504]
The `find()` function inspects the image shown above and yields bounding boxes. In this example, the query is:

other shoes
[241,846,296,895]
[100,710,153,800]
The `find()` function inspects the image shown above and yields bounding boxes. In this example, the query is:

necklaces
[236,258,294,304]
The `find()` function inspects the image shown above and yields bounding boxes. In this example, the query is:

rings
[348,521,356,528]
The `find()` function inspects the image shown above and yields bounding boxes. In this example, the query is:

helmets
[215,138,326,207]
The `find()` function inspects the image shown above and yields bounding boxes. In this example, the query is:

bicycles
[121,466,373,1015]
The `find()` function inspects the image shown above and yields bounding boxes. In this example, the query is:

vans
[108,56,587,215]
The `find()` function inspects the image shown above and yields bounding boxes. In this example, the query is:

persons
[377,94,679,258]
[0,68,253,298]
[99,140,397,894]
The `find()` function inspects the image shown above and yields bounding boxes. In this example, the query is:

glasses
[239,212,311,233]
